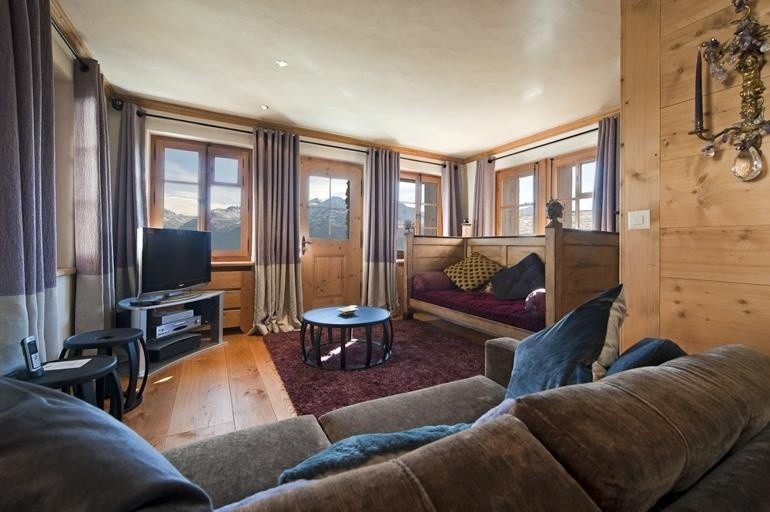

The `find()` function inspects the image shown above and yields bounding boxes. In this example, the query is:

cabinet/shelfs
[119,289,232,377]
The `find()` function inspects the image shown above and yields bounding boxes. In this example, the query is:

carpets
[261,314,497,417]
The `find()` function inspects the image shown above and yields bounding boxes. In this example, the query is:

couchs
[399,216,619,347]
[0,338,770,512]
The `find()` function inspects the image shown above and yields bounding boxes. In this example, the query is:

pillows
[487,285,626,403]
[493,252,546,302]
[272,417,471,472]
[444,251,502,292]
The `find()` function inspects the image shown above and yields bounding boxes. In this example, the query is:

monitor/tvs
[136,227,211,304]
